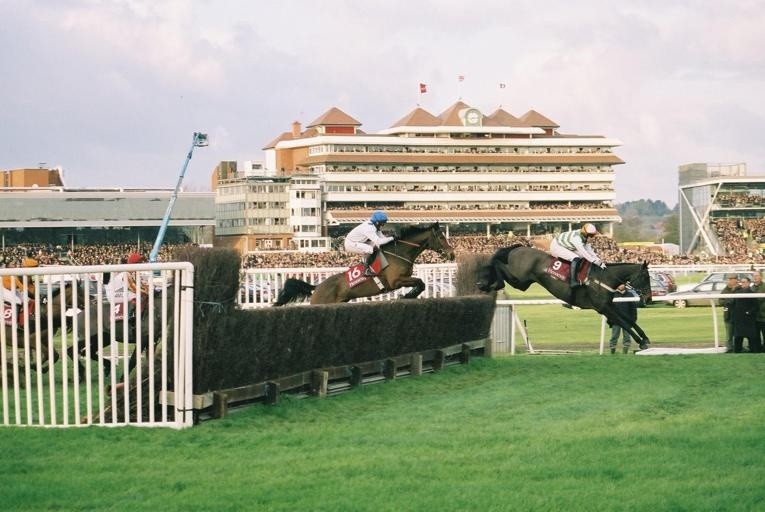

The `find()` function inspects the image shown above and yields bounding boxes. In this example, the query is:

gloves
[600,263,607,270]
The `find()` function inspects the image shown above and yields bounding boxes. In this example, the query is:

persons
[749,270,765,353]
[549,222,607,289]
[343,211,403,277]
[619,245,765,265]
[709,188,765,244]
[1,255,49,335]
[244,249,360,269]
[718,273,747,354]
[100,251,171,327]
[415,228,619,267]
[606,284,638,354]
[0,240,197,267]
[724,277,761,354]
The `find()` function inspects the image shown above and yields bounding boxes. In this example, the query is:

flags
[500,83,506,90]
[458,73,465,83]
[419,82,429,95]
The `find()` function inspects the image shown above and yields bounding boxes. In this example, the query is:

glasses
[588,234,594,238]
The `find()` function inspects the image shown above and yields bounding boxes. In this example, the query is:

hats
[727,274,737,279]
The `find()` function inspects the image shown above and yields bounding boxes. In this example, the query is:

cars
[646,271,756,310]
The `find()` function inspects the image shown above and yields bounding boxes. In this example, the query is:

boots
[363,254,376,276]
[569,261,585,288]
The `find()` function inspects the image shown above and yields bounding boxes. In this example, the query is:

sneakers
[623,349,627,354]
[611,348,615,353]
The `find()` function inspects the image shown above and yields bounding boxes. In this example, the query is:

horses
[274,222,456,306]
[29,283,174,383]
[476,245,649,350]
[5,279,94,374]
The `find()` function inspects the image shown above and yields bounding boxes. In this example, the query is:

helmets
[370,211,388,223]
[581,223,597,238]
[128,253,145,264]
[23,259,39,267]
